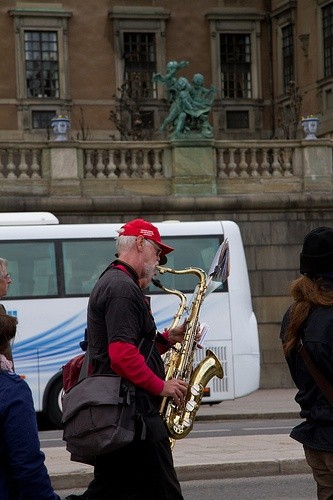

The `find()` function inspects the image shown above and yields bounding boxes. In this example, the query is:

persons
[159,60,213,135]
[1,259,16,372]
[1,314,62,500]
[280,226,333,500]
[65,218,203,500]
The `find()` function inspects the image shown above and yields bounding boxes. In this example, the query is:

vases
[301,118,319,139]
[52,117,70,140]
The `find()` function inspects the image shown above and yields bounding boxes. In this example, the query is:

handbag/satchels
[62,373,138,457]
[62,353,93,392]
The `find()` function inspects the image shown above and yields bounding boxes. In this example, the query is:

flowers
[301,114,319,119]
[48,113,68,119]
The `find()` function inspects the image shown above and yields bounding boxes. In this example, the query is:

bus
[0,211,261,432]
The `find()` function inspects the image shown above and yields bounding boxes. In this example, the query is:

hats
[118,218,174,256]
[299,226,333,274]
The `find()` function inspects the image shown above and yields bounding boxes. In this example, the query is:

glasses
[3,274,10,280]
[144,238,162,256]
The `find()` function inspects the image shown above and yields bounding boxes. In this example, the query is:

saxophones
[151,277,187,415]
[155,266,224,441]
